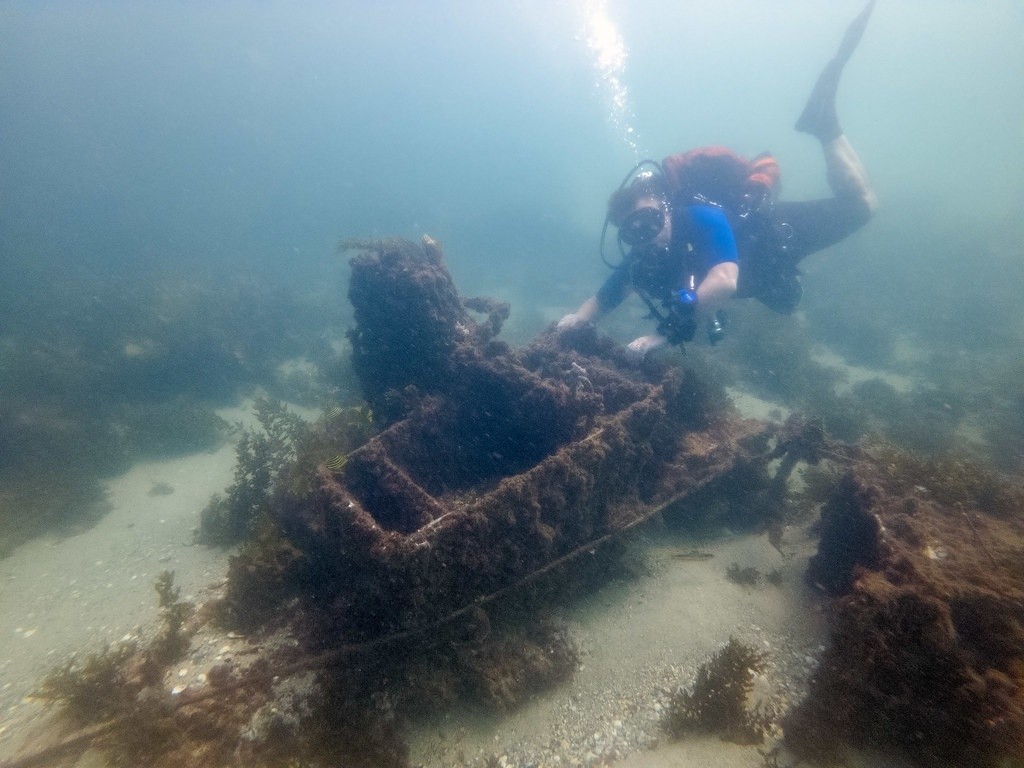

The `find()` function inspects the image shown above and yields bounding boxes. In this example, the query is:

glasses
[618,205,665,246]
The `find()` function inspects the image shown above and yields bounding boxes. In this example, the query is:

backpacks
[662,145,779,220]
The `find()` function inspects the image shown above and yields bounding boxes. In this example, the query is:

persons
[556,0,877,370]
